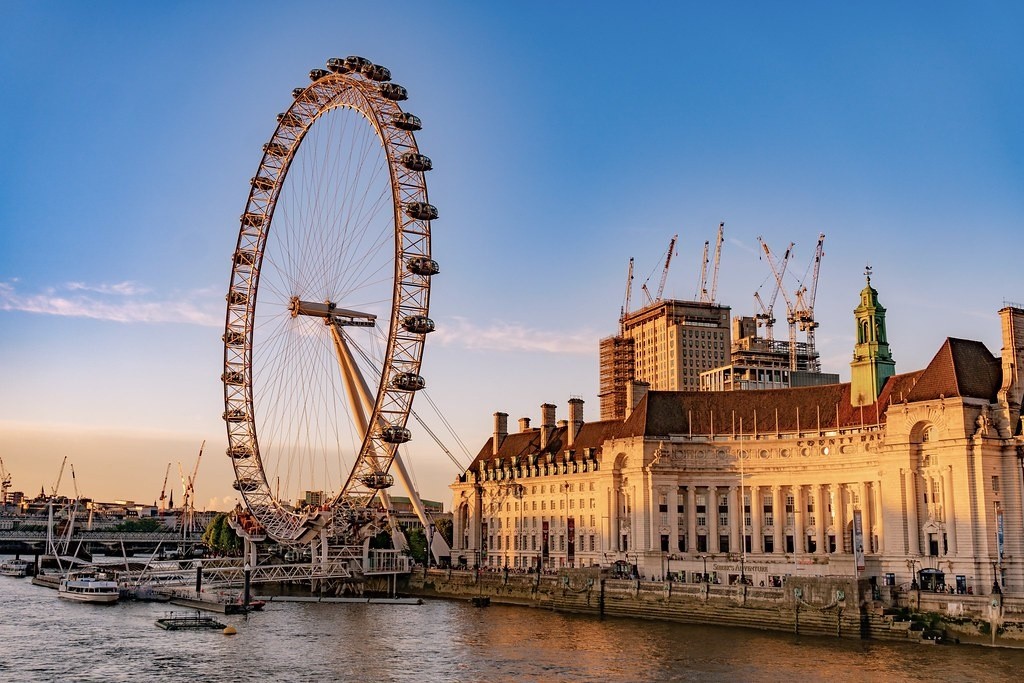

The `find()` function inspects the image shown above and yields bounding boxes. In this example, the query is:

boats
[0,558,32,577]
[57,577,122,605]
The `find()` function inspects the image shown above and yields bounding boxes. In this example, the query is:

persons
[612,571,790,591]
[412,560,559,576]
[933,582,973,593]
[232,512,266,535]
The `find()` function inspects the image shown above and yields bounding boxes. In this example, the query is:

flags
[542,520,549,562]
[854,509,866,572]
[567,517,575,562]
[996,510,1004,568]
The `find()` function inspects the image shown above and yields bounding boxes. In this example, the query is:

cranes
[176,438,207,509]
[157,462,174,513]
[69,463,84,499]
[696,219,726,306]
[751,230,827,372]
[0,457,13,504]
[49,455,68,496]
[619,233,680,316]
